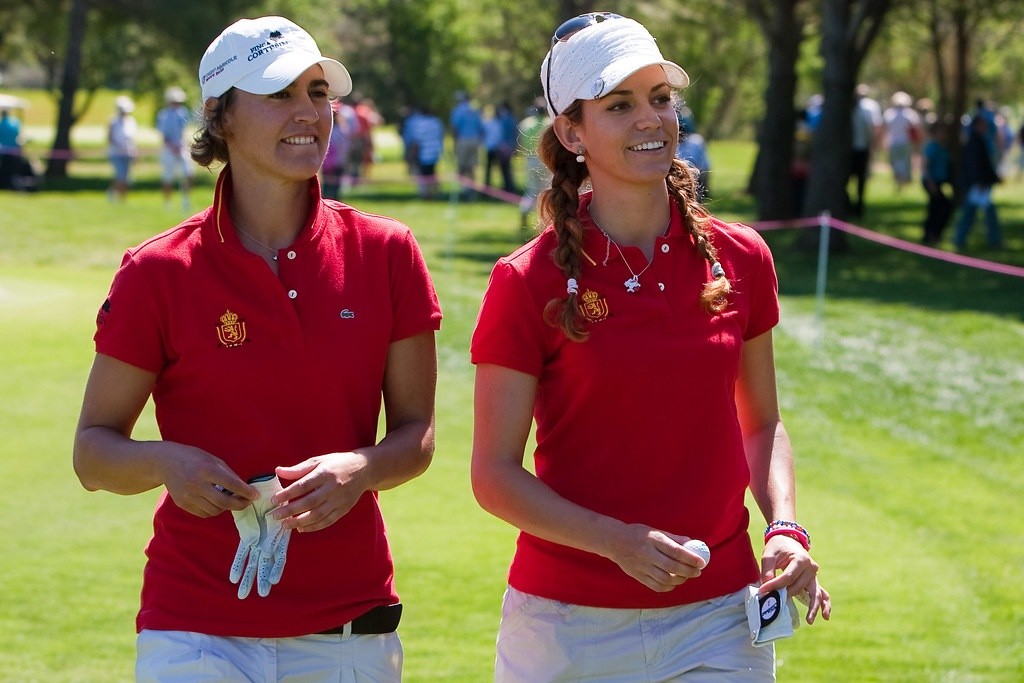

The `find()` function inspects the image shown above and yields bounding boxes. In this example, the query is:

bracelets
[764,520,811,552]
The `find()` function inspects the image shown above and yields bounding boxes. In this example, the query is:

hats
[540,18,691,122]
[116,95,134,113]
[199,15,351,104]
[164,86,186,102]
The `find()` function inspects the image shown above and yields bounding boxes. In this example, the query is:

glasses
[546,11,622,117]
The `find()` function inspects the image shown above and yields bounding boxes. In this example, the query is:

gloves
[227,473,294,600]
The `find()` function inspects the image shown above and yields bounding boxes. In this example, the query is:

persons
[156,86,190,210]
[73,17,443,683]
[106,95,139,205]
[471,13,832,683]
[319,84,1024,257]
[0,110,19,189]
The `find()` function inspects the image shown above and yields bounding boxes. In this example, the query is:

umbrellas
[0,94,27,125]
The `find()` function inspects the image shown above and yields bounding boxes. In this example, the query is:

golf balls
[681,540,712,570]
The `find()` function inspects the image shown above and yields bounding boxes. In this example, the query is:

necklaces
[231,219,279,262]
[587,204,672,293]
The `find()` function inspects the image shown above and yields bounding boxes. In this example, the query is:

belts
[313,602,403,636]
